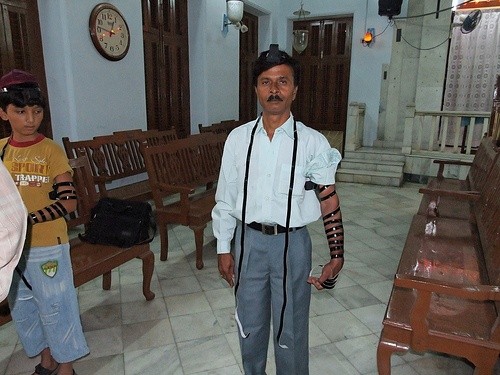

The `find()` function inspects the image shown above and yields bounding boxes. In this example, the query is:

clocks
[90,3,131,61]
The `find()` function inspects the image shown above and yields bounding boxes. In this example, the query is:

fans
[449,10,482,37]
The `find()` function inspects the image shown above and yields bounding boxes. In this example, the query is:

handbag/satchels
[78,198,157,247]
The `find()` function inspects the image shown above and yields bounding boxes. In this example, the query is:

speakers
[378,0,403,16]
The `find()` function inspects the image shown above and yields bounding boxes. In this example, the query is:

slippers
[58,369,75,375]
[31,363,59,375]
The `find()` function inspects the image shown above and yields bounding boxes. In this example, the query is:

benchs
[377,136,500,375]
[0,120,244,326]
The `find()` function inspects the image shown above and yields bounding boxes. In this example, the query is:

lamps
[362,32,373,47]
[223,1,248,33]
[293,0,310,54]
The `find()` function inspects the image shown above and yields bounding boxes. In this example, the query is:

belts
[248,221,302,235]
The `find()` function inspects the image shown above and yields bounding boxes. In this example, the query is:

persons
[211,50,345,375]
[0,70,90,375]
[0,157,27,303]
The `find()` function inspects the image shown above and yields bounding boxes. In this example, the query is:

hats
[257,51,294,63]
[0,70,41,90]
